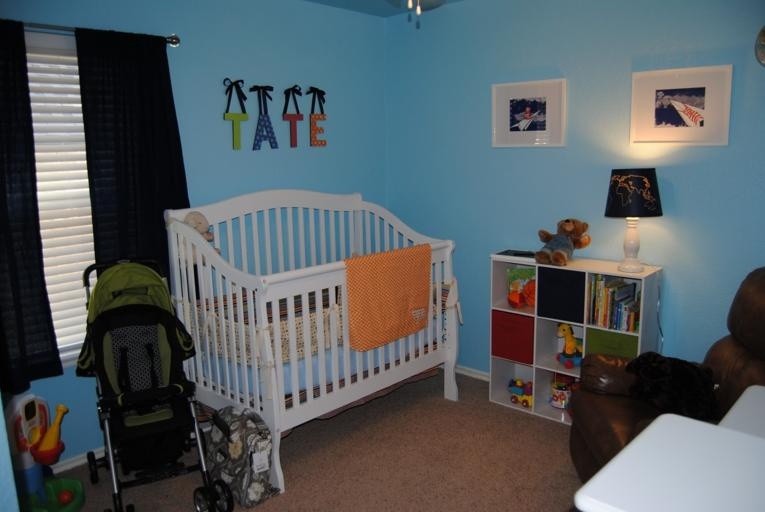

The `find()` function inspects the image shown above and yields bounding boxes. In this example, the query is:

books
[590,274,641,334]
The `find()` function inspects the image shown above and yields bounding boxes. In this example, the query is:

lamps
[604,166,663,274]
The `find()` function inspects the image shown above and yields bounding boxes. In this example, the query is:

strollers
[75,261,234,512]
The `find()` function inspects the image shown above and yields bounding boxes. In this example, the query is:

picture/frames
[492,80,566,148]
[631,64,733,146]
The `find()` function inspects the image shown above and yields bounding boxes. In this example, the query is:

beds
[165,189,462,495]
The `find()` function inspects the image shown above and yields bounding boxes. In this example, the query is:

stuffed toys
[534,218,591,266]
[184,212,221,264]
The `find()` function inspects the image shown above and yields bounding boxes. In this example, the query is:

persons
[655,95,684,128]
[523,107,532,119]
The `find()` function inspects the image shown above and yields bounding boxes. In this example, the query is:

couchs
[566,267,765,484]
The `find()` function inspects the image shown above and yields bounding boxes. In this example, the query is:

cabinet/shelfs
[490,251,663,426]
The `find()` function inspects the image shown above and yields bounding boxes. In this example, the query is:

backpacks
[206,403,281,509]
